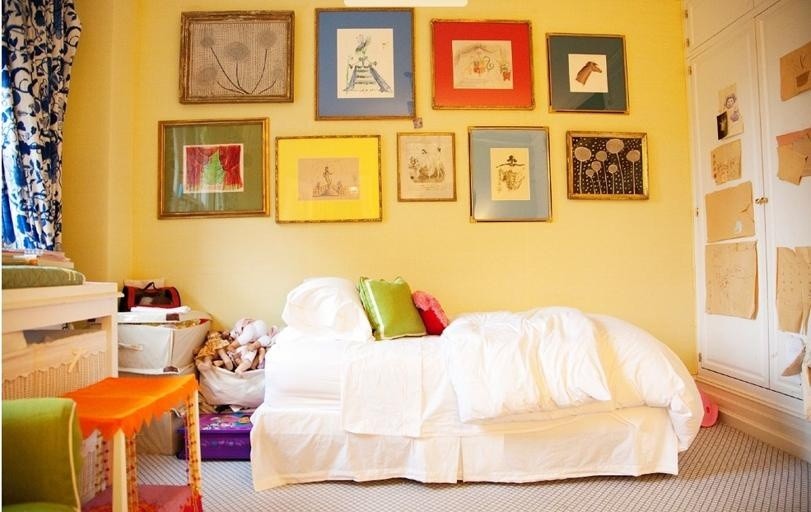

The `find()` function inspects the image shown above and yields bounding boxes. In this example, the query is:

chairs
[3,396,86,511]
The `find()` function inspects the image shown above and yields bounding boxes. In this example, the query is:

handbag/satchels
[119,282,181,312]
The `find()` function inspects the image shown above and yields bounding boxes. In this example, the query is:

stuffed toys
[193,317,277,374]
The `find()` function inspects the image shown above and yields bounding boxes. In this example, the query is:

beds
[249,321,703,488]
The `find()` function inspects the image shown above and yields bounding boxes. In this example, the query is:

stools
[64,372,212,512]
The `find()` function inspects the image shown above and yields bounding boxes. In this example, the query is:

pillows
[277,275,451,342]
